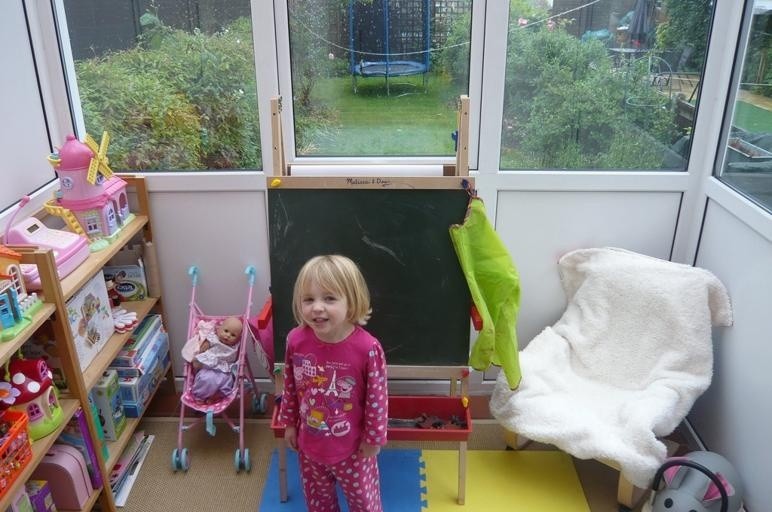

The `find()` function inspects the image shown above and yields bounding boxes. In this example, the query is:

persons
[181,318,242,406]
[276,254,390,512]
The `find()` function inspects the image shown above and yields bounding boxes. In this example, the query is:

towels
[489,244,732,491]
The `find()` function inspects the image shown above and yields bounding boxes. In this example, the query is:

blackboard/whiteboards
[267,176,475,379]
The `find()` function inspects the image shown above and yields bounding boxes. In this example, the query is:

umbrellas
[627,0,651,47]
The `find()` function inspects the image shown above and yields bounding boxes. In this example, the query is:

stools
[504,250,707,509]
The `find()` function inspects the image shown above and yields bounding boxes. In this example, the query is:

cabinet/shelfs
[1,175,177,510]
